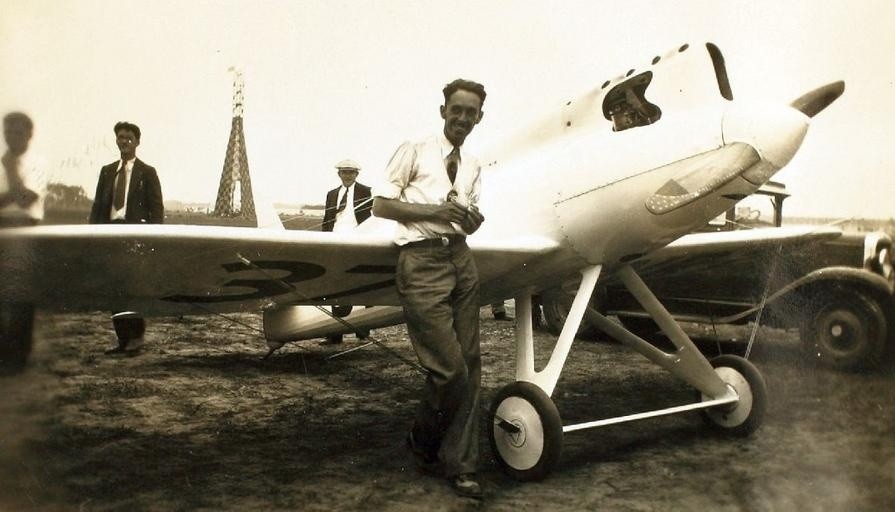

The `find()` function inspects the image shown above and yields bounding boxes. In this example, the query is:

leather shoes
[405,426,443,471]
[447,467,484,499]
[107,336,147,360]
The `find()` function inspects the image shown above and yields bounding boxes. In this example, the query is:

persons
[0,112,46,378]
[318,159,372,345]
[88,121,164,355]
[370,78,486,498]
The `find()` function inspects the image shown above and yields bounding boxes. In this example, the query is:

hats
[335,159,362,171]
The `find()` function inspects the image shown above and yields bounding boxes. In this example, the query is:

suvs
[542,179,894,371]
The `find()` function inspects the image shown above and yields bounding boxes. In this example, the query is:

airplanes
[1,34,895,493]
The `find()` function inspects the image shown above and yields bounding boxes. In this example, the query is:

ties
[110,160,131,207]
[338,185,352,214]
[442,145,468,187]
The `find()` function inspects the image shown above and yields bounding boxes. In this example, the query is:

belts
[404,232,468,252]
